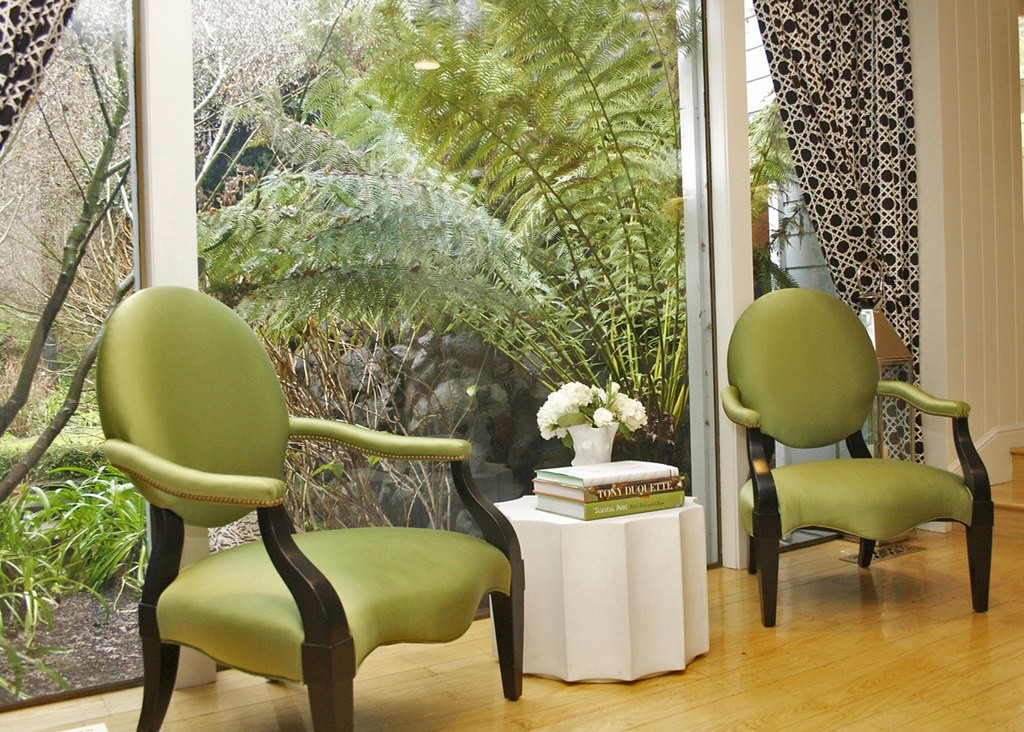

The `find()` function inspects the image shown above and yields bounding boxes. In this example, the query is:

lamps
[857,260,914,548]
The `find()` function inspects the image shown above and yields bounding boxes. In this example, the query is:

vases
[567,422,619,465]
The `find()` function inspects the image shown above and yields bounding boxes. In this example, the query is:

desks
[488,494,710,683]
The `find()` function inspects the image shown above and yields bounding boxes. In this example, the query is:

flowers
[536,373,647,448]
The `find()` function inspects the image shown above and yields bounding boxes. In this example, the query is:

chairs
[720,287,994,625]
[97,286,525,732]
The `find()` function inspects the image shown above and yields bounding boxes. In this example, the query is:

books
[533,460,685,521]
